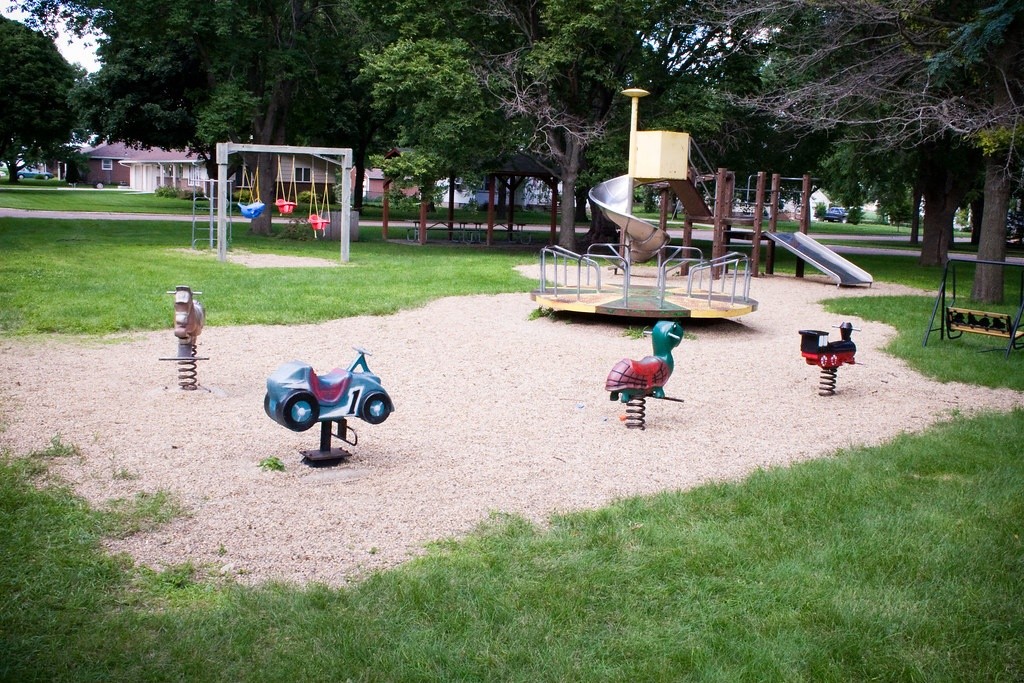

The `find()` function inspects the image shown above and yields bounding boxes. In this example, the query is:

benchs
[406,228,532,244]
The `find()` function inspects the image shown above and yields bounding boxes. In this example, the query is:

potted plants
[814,202,828,222]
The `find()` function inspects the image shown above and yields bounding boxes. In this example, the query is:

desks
[409,220,530,241]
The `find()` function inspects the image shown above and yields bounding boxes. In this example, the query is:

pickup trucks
[824,206,849,224]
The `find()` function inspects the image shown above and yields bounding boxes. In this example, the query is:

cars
[16,168,54,180]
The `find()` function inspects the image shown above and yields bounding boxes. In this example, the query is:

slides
[761,230,875,289]
[587,173,671,264]
